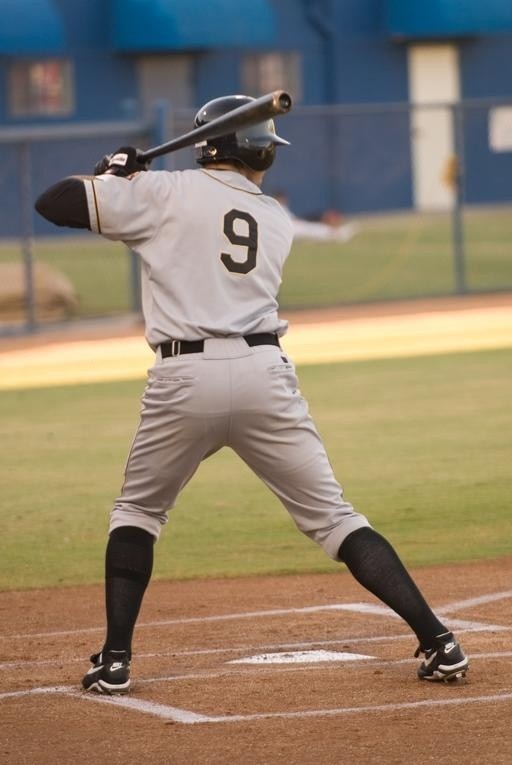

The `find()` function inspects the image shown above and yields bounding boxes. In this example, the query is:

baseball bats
[138,90,291,161]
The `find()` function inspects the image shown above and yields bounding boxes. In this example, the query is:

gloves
[94,146,152,177]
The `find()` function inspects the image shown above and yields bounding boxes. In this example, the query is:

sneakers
[414,632,470,683]
[82,650,130,695]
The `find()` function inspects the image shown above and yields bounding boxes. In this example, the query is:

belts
[161,333,280,358]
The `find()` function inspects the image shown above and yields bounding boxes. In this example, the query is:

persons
[36,94,470,693]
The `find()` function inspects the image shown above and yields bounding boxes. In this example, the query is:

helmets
[193,95,291,171]
